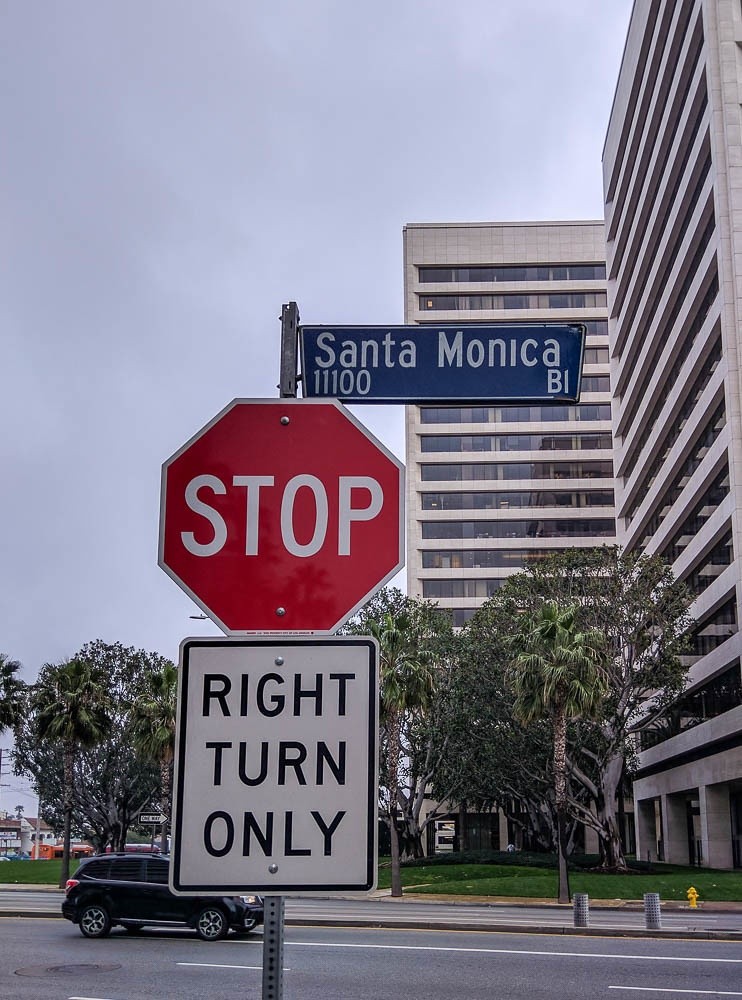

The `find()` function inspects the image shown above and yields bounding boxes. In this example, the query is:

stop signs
[157,398,405,638]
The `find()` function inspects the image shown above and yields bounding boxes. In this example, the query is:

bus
[31,844,94,860]
[106,843,161,855]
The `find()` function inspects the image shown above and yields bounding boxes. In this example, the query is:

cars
[0,851,31,862]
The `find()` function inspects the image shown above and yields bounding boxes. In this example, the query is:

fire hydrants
[687,887,699,908]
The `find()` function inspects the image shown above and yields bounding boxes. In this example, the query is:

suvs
[62,852,266,942]
